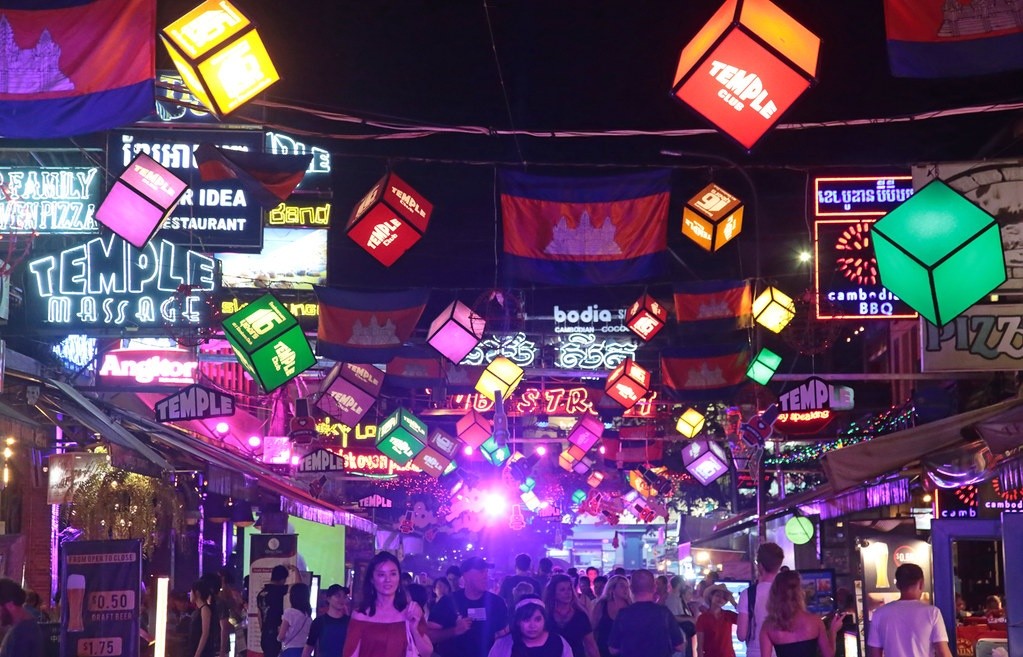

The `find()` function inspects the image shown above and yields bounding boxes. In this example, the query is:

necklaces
[554,608,572,619]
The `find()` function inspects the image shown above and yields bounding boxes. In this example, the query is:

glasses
[754,559,762,565]
[187,592,193,597]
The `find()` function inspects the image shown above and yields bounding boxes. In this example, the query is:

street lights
[659,148,766,547]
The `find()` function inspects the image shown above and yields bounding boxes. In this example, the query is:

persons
[866,563,952,657]
[954,567,1005,618]
[0,578,50,657]
[737,542,847,657]
[187,551,738,657]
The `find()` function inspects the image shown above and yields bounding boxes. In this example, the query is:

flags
[312,287,447,388]
[0,0,158,139]
[882,0,1023,79]
[494,164,673,289]
[601,280,753,470]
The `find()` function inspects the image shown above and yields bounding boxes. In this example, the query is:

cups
[66,574,86,632]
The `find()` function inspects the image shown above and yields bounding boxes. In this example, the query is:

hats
[460,556,496,576]
[326,584,350,596]
[552,567,565,573]
[702,584,733,608]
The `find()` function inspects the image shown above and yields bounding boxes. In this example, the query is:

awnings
[81,394,379,537]
[655,397,1023,561]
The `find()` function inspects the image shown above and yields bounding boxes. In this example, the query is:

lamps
[216,421,232,440]
[247,435,262,459]
[858,534,869,548]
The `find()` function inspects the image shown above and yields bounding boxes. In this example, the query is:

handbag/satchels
[676,614,697,638]
[405,601,420,657]
